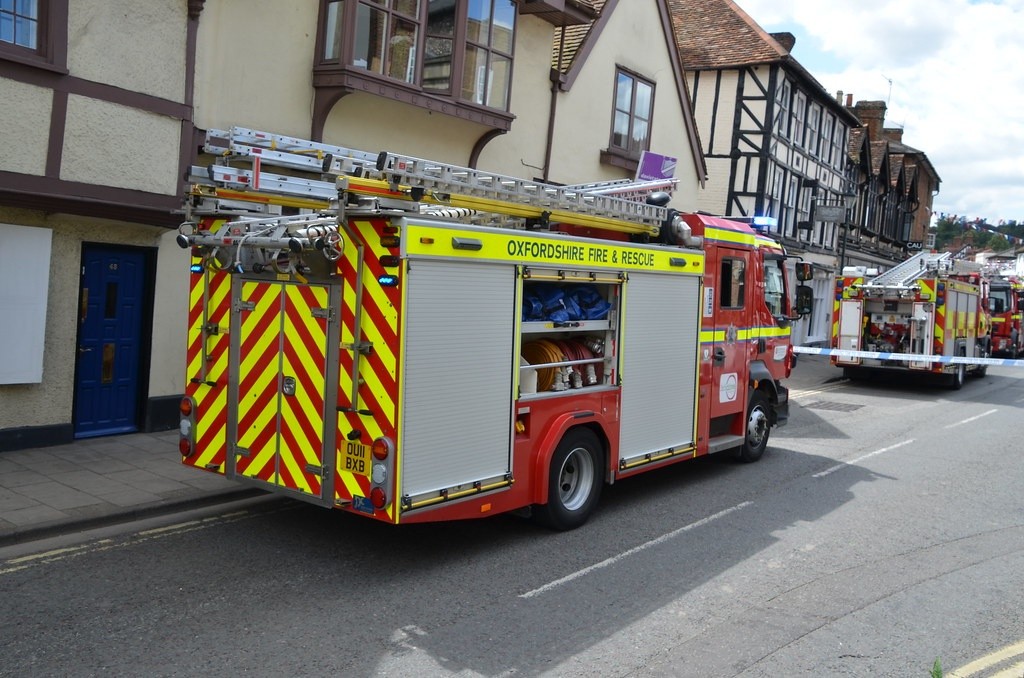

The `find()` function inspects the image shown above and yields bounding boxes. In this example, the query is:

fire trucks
[830,250,1004,390]
[988,274,1024,359]
[175,124,815,532]
